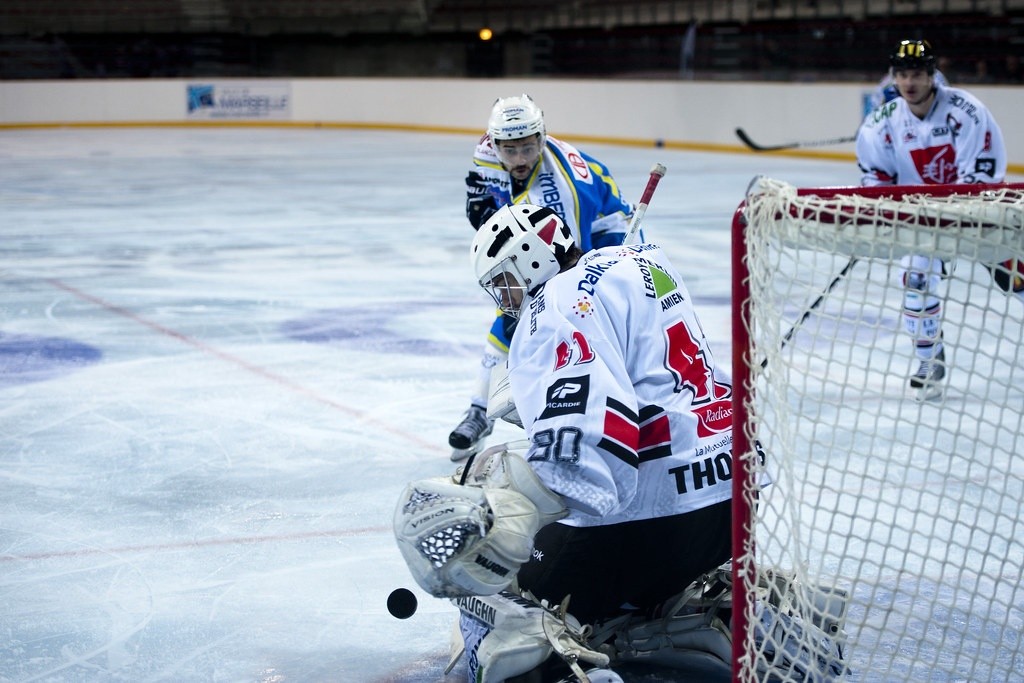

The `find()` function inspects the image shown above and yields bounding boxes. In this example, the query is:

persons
[448,94,644,448]
[393,203,766,623]
[854,36,1024,402]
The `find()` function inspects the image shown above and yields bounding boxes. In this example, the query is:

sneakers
[910,348,945,402]
[449,404,495,462]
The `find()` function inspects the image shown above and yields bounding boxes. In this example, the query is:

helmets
[890,39,938,67]
[488,93,547,162]
[470,204,575,320]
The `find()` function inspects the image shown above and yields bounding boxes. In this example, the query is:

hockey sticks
[735,126,856,152]
[617,160,668,248]
[751,257,858,380]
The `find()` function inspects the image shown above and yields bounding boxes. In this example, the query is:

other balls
[387,588,418,620]
